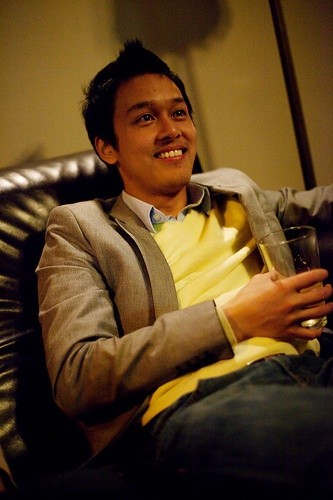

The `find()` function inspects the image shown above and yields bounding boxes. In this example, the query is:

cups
[260,227,328,329]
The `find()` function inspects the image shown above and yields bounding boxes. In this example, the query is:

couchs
[0,149,205,500]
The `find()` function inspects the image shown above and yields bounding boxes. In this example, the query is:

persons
[34,37,333,500]
